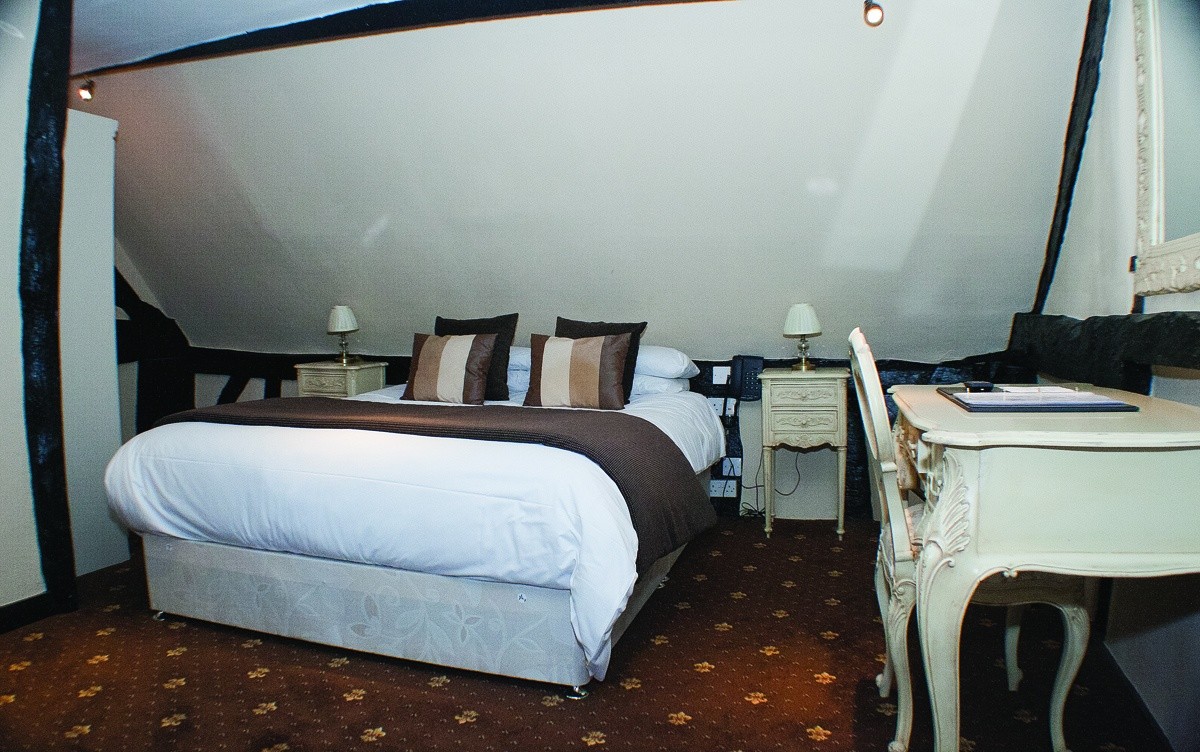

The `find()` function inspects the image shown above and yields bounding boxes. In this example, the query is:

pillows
[506,368,692,400]
[507,341,700,380]
[435,313,520,400]
[400,333,498,401]
[521,334,632,409]
[553,314,649,403]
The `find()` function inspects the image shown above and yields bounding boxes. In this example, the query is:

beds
[101,375,729,702]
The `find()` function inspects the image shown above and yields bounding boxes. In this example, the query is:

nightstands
[295,360,392,396]
[755,366,852,543]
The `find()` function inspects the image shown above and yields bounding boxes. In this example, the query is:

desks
[881,381,1200,752]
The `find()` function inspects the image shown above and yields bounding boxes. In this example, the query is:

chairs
[844,326,1097,752]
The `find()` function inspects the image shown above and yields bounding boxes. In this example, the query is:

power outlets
[708,396,738,416]
[708,479,738,497]
[722,456,743,477]
[713,366,732,385]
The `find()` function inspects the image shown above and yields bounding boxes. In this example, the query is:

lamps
[327,304,363,366]
[782,301,824,373]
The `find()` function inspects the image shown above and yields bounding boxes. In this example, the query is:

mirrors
[1132,0,1200,298]
